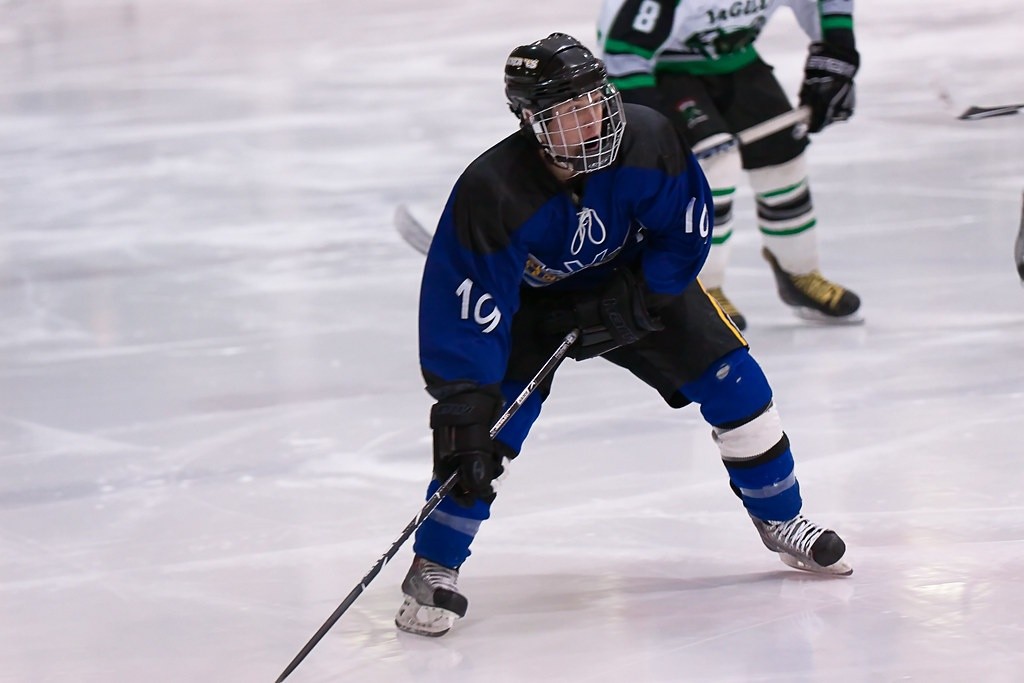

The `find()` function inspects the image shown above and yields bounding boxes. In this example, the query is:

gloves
[426,380,500,508]
[792,41,859,141]
[561,284,666,362]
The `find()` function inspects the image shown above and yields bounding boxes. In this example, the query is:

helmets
[505,32,626,173]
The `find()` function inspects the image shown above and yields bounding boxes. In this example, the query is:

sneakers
[745,508,853,575]
[396,554,468,638]
[708,287,748,332]
[763,244,860,325]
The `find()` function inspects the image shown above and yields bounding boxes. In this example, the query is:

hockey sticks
[274,325,582,682]
[391,103,815,257]
[935,79,1023,122]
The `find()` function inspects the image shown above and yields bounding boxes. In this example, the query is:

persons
[394,32,853,638]
[597,0,866,326]
